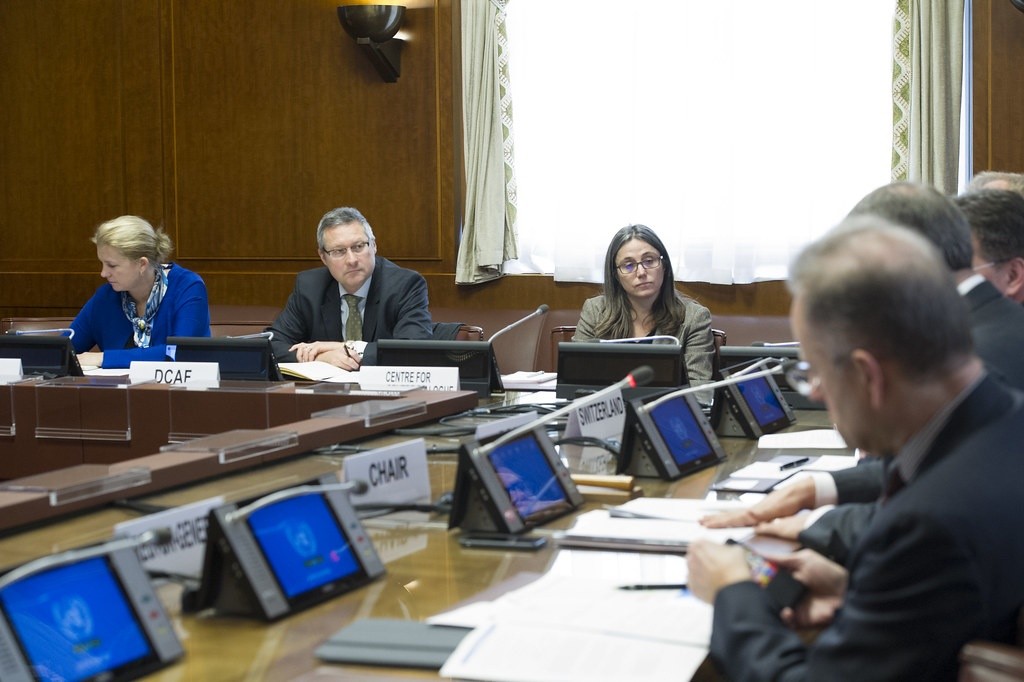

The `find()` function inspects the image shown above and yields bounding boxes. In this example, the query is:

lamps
[336,5,406,84]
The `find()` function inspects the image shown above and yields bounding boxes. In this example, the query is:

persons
[267,208,433,371]
[685,172,1024,682]
[59,215,212,369]
[570,223,715,408]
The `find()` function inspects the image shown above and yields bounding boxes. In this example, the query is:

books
[499,370,557,392]
[278,360,343,381]
[437,455,864,682]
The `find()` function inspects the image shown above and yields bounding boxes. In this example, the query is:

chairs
[0,318,101,353]
[209,320,272,338]
[431,323,485,342]
[551,326,727,374]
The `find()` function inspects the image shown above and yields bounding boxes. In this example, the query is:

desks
[0,373,850,682]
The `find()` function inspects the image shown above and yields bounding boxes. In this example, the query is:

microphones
[587,335,679,345]
[0,530,172,589]
[637,355,800,415]
[487,304,549,345]
[221,332,273,341]
[226,480,369,524]
[6,327,75,340]
[751,341,800,348]
[473,365,655,458]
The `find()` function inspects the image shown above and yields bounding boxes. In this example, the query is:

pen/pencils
[780,457,809,472]
[344,345,350,357]
[617,584,688,589]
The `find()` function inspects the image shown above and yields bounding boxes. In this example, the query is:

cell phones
[458,533,546,548]
[725,538,811,616]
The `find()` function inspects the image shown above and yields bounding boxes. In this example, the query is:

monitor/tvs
[0,335,84,376]
[472,426,584,534]
[223,483,386,623]
[375,339,505,395]
[167,337,284,381]
[717,358,796,438]
[556,342,691,389]
[716,347,802,391]
[0,539,184,682]
[629,383,727,480]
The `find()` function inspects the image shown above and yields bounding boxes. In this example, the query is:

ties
[342,294,365,341]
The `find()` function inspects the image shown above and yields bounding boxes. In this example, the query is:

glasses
[785,350,858,395]
[616,255,664,274]
[323,238,371,258]
